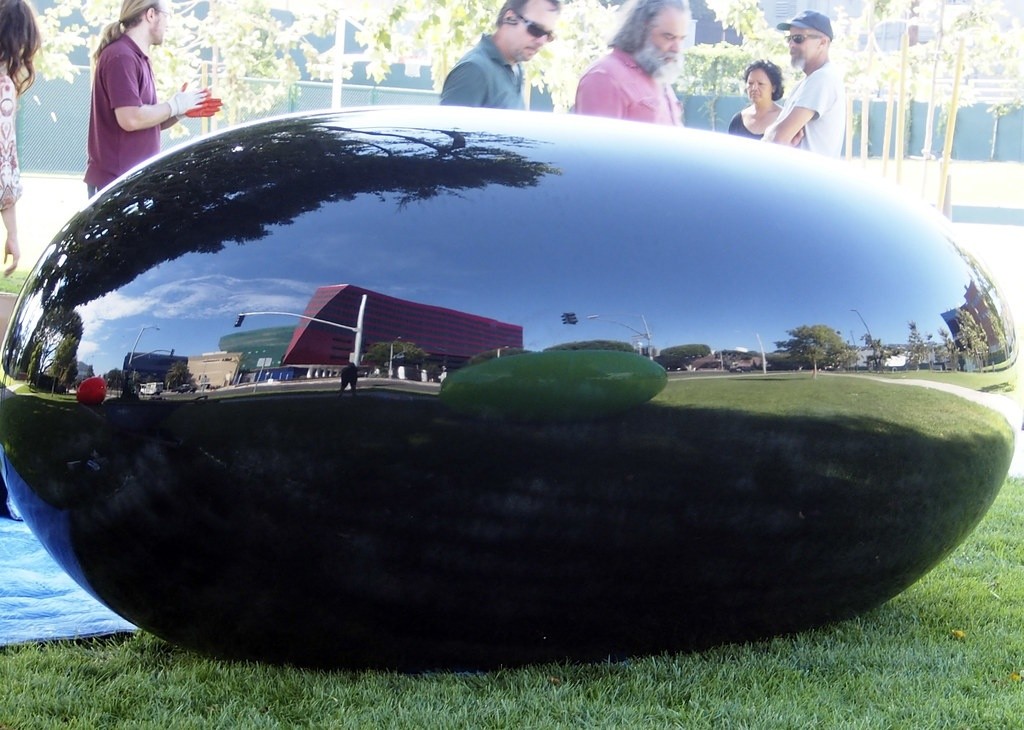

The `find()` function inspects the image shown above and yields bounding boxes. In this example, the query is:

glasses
[158,10,173,19]
[784,35,823,43]
[517,12,557,43]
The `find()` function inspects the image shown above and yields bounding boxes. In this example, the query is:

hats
[777,10,834,42]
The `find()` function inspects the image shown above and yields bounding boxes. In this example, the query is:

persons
[338,362,359,398]
[438,0,562,111]
[727,11,846,159]
[1,0,45,522]
[82,2,223,199]
[571,0,689,127]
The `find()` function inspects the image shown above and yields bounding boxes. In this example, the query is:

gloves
[166,82,211,118]
[174,92,223,120]
